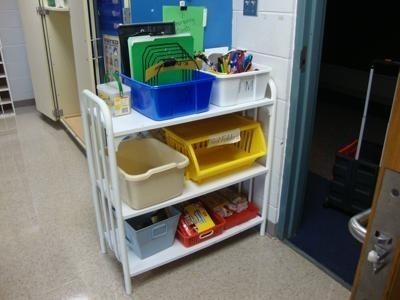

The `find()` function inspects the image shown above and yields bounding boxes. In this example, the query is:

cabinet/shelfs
[0,46,16,119]
[80,76,277,295]
[18,0,103,151]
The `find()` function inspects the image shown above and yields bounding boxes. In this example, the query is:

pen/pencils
[218,50,253,73]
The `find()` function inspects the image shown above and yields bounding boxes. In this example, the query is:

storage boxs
[115,68,271,260]
